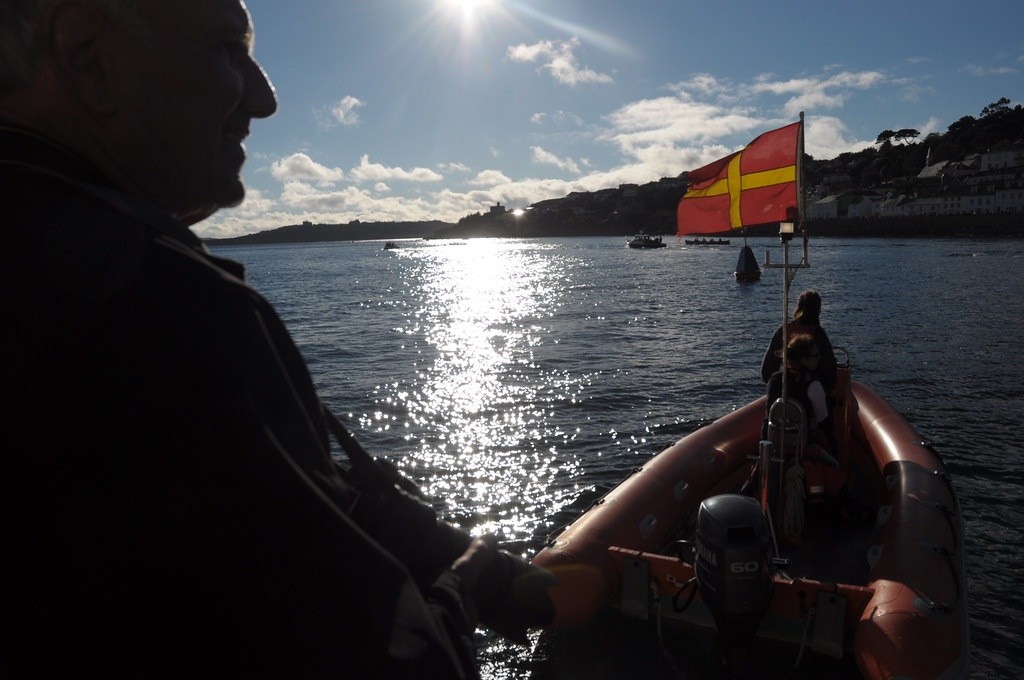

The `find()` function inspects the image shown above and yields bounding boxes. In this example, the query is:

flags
[676,121,801,235]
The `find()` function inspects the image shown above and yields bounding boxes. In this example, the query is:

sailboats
[736,235,761,281]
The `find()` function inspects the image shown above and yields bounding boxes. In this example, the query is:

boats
[686,238,730,244]
[627,233,666,248]
[528,108,967,679]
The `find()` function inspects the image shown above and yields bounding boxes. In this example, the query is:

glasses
[806,352,820,359]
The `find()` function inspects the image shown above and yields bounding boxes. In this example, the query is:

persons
[0,0,498,680]
[761,292,836,445]
